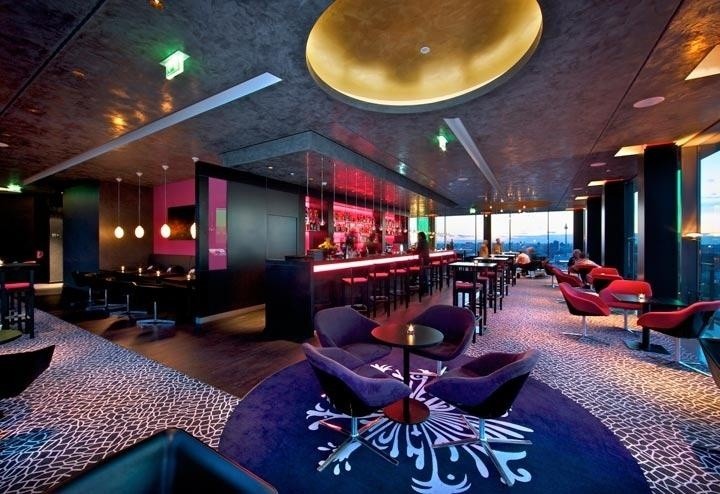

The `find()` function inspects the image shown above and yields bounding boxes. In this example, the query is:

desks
[371,322,445,424]
[447,251,517,344]
[0,259,40,331]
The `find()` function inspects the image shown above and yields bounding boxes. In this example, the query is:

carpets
[0,295,241,494]
[218,354,652,493]
[450,286,720,494]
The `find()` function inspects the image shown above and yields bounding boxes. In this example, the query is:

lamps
[435,136,448,151]
[306,152,436,235]
[114,176,125,240]
[134,171,145,239]
[190,156,199,239]
[160,164,171,239]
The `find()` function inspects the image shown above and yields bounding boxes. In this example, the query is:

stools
[71,270,176,329]
[4,282,31,334]
[342,252,463,318]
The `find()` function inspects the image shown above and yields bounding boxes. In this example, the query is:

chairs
[404,305,477,377]
[313,307,392,364]
[302,343,412,472]
[424,348,542,488]
[517,256,720,355]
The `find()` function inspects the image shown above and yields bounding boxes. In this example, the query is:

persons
[493,238,501,253]
[514,252,530,275]
[479,239,488,258]
[411,232,429,294]
[361,233,382,257]
[568,249,588,284]
